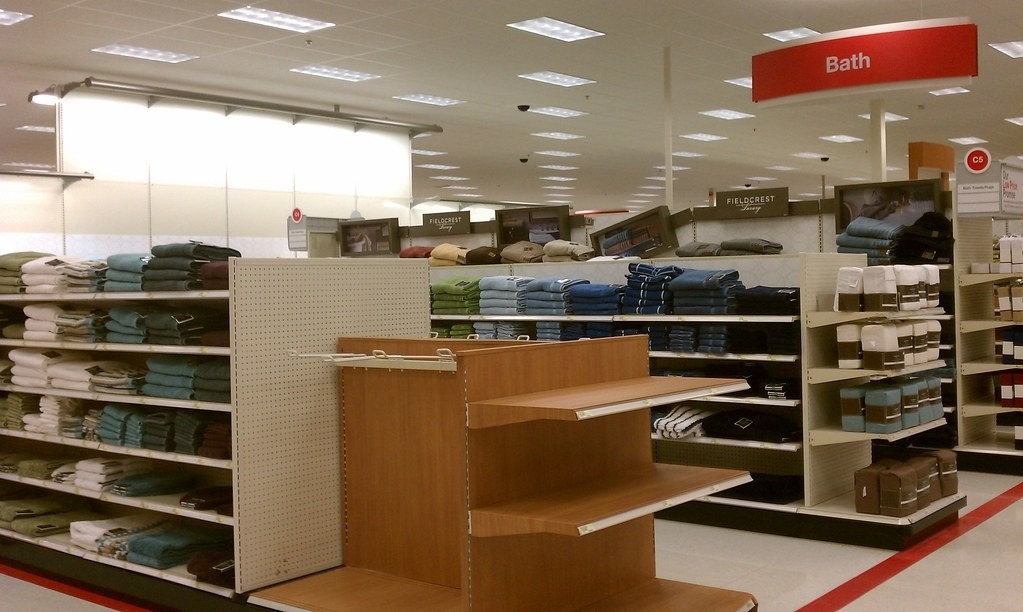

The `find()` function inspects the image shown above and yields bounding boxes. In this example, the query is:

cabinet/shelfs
[0,167,1023,612]
